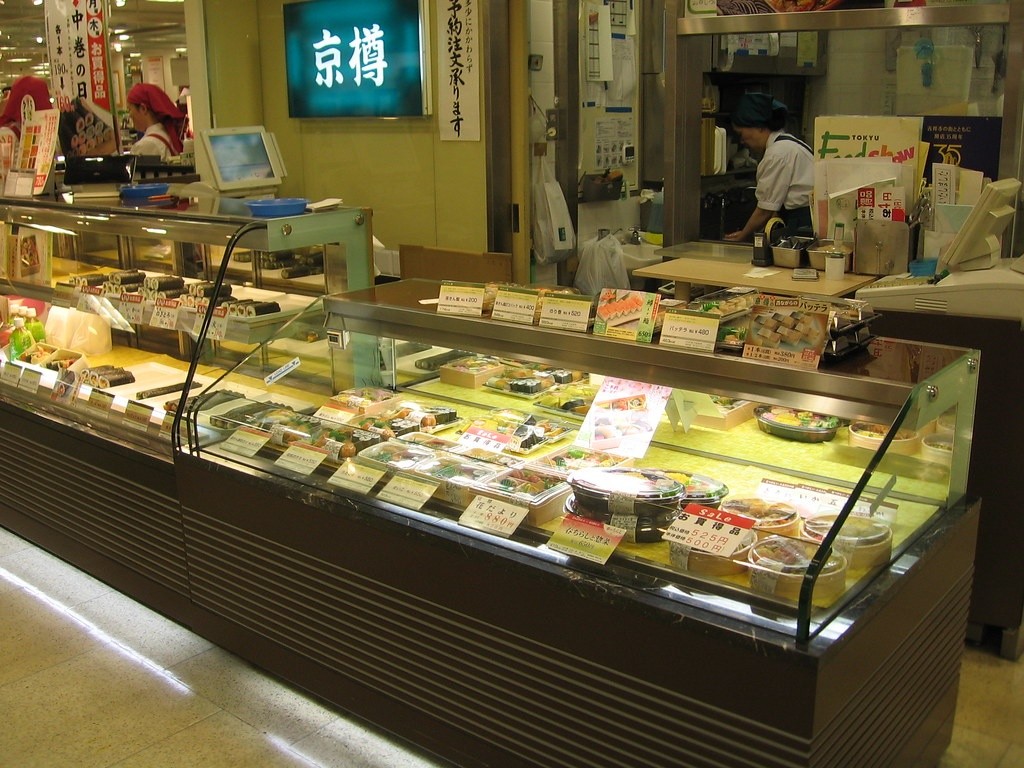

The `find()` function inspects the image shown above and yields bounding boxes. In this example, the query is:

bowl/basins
[565,464,729,543]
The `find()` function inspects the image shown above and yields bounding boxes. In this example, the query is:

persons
[724,101,814,245]
[0,77,63,162]
[127,83,185,160]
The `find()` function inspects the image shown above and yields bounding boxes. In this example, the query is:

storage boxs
[438,355,505,388]
[0,223,88,394]
[3,168,36,198]
[680,395,762,428]
[331,385,402,418]
[355,431,636,528]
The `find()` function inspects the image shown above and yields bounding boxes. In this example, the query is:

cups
[825,255,845,279]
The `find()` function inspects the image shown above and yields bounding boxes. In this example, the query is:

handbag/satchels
[572,233,631,296]
[531,156,576,264]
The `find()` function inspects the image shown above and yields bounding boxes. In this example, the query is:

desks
[631,259,876,304]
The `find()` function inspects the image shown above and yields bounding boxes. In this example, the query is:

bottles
[7,304,47,363]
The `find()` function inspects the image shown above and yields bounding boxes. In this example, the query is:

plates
[244,197,311,216]
[121,183,170,198]
[753,406,842,443]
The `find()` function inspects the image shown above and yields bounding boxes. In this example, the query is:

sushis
[68,268,282,318]
[57,101,112,157]
[135,381,292,429]
[80,364,134,389]
[231,249,325,279]
[395,342,585,413]
[249,387,552,455]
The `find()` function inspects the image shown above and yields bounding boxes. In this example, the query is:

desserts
[597,295,643,321]
[752,311,821,348]
